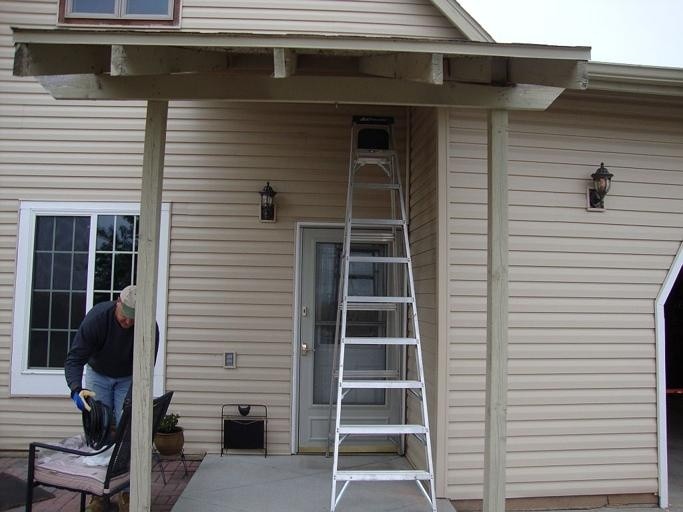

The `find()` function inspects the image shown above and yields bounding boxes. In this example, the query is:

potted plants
[157,415,184,455]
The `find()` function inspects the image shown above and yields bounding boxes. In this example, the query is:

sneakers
[119,491,128,512]
[85,495,110,512]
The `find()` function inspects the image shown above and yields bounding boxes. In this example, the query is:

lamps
[259,181,278,221]
[586,162,614,211]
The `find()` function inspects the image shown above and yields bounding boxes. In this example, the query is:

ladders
[326,122,437,511]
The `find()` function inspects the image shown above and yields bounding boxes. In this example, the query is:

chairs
[24,390,174,512]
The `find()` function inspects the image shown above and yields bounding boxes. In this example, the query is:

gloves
[70,388,96,412]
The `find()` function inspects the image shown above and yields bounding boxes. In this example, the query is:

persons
[63,286,160,511]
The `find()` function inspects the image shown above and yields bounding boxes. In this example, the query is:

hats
[119,284,136,320]
